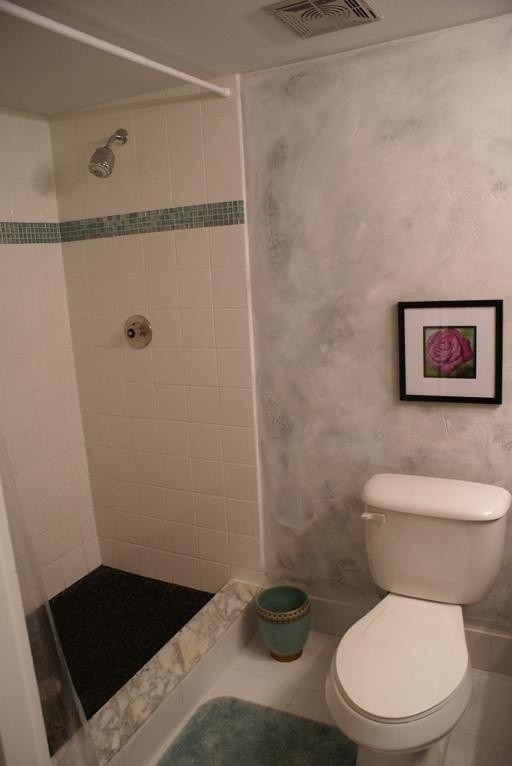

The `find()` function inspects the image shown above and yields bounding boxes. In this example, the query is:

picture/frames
[397,298,503,405]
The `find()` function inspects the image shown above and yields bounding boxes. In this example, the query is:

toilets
[326,472,512,766]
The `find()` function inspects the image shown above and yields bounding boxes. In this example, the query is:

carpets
[154,696,357,765]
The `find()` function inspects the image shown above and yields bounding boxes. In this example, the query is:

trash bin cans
[254,585,311,662]
